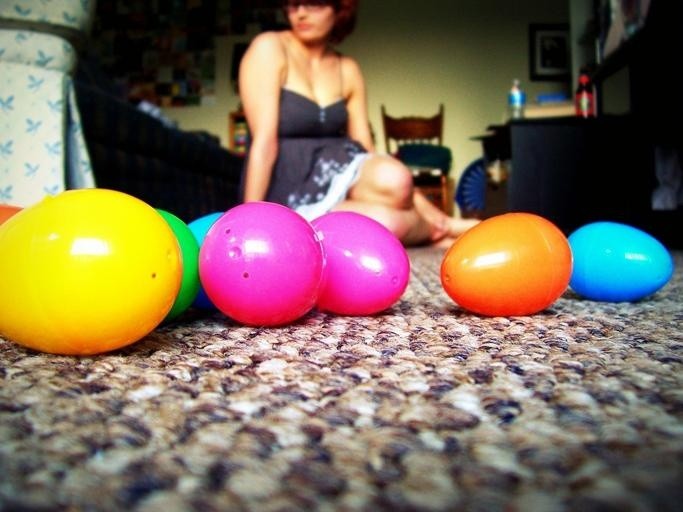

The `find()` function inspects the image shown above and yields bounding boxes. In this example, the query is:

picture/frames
[527,21,572,83]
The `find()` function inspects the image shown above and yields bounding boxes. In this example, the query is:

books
[522,101,577,121]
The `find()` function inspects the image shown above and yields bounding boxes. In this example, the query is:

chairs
[380,104,451,217]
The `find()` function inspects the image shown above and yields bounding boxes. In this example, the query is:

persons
[233,0,482,247]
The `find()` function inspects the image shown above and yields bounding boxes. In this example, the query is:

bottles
[574,75,594,118]
[506,77,526,122]
